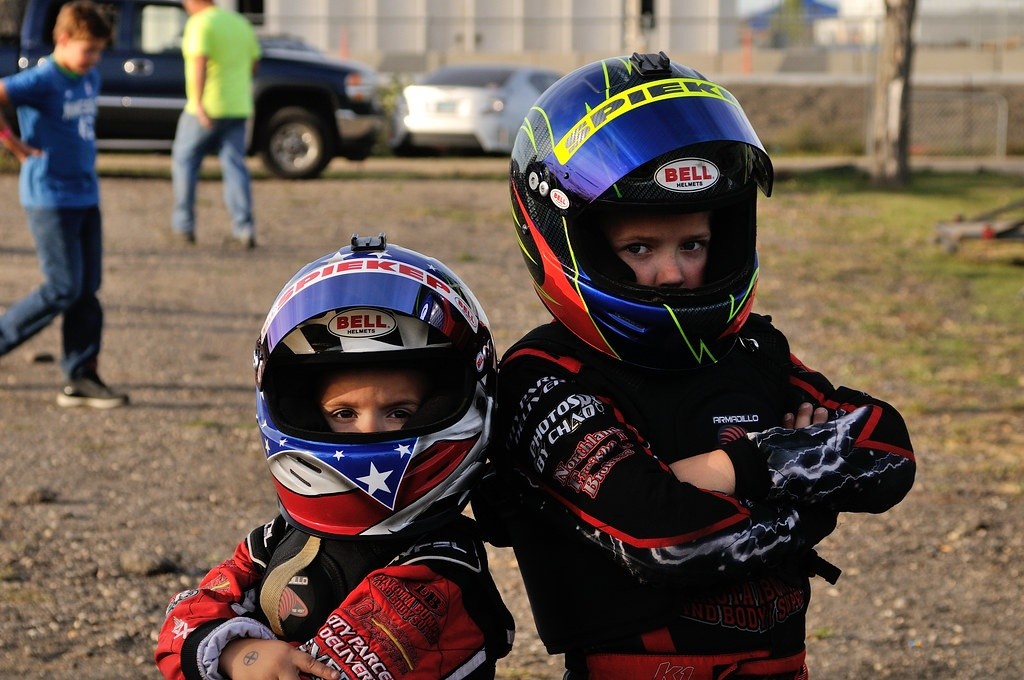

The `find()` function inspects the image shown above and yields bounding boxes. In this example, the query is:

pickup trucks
[0,2,381,180]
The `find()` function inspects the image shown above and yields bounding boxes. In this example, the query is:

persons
[0,0,129,409]
[157,236,515,680]
[169,0,263,249]
[498,51,916,680]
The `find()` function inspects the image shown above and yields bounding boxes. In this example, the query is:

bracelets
[0,127,12,140]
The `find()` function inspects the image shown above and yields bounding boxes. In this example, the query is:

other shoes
[57,371,129,409]
[214,233,256,253]
[155,230,200,250]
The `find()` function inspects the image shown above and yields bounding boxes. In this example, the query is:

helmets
[251,231,502,544]
[508,51,777,376]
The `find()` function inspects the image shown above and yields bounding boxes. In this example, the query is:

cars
[386,60,571,156]
